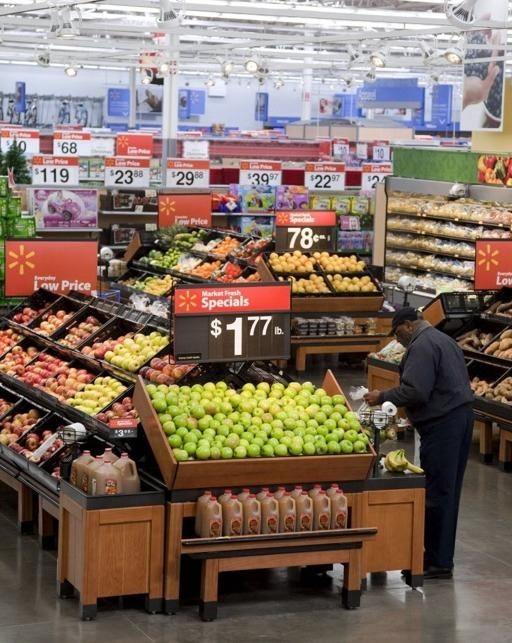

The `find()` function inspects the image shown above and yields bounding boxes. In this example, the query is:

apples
[144,380,369,459]
[0,306,215,461]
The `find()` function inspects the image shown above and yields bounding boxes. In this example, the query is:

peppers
[477,154,512,187]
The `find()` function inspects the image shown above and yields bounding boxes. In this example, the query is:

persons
[362,306,477,582]
[461,12,499,113]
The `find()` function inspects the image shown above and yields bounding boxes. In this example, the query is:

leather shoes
[401,566,452,579]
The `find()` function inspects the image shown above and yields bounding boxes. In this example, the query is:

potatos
[458,300,512,406]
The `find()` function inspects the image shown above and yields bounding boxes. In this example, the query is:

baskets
[1,287,209,470]
[263,248,384,298]
[113,223,275,310]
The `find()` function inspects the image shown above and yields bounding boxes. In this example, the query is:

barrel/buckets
[194,483,349,538]
[70,447,140,496]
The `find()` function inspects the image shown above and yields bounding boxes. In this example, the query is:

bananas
[384,449,426,474]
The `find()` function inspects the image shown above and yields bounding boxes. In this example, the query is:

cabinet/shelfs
[385,210,511,283]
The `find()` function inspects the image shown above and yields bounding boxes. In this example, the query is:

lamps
[36,49,51,67]
[346,43,361,64]
[64,64,77,77]
[427,67,443,84]
[369,46,391,68]
[244,56,262,73]
[56,22,77,39]
[445,38,466,63]
[157,1,177,23]
[364,68,378,83]
[139,69,152,85]
[446,0,478,28]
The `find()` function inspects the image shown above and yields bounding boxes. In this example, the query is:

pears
[268,250,378,294]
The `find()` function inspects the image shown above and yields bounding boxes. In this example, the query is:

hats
[386,307,423,337]
